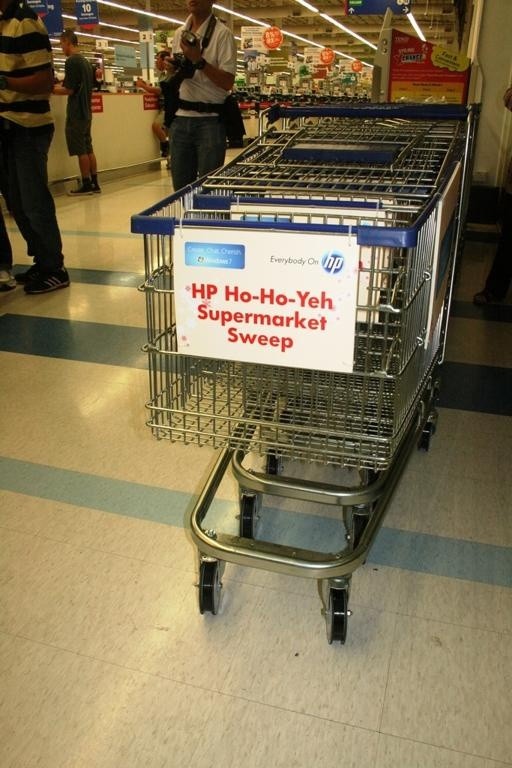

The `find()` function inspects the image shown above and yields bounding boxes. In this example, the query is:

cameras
[166,52,194,90]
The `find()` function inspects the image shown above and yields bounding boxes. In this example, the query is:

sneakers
[15,263,43,284]
[160,140,170,158]
[68,187,93,196]
[91,185,101,193]
[473,287,506,306]
[0,270,14,292]
[24,265,71,294]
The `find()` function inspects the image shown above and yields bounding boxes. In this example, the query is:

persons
[0,0,70,294]
[53,30,101,195]
[164,0,237,192]
[136,51,173,168]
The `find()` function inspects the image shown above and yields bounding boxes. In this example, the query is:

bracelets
[193,59,207,70]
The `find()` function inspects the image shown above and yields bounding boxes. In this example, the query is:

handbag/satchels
[159,73,187,127]
[219,95,246,150]
